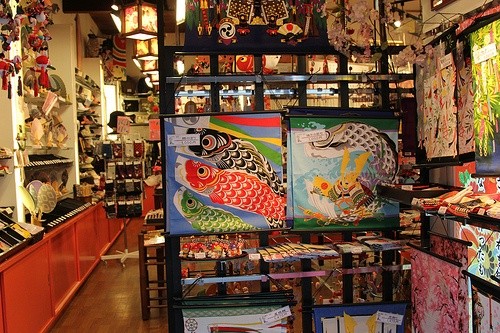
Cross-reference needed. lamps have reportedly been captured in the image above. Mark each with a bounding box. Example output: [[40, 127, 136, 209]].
[[117, 0, 158, 42], [132, 35, 159, 61], [140, 59, 160, 95]]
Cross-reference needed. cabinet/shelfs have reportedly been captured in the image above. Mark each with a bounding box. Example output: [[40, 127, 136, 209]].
[[158, 0, 499, 333], [1, 0, 136, 332]]
